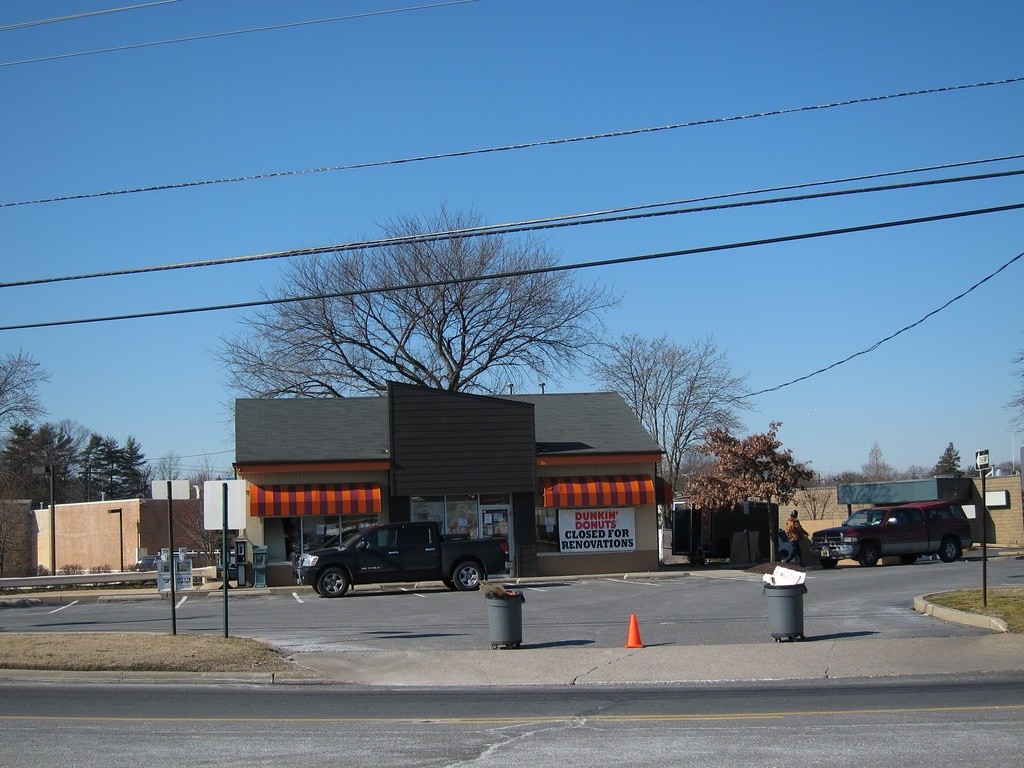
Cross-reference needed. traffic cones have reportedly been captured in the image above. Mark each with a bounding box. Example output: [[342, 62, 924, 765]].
[[625, 614, 644, 648]]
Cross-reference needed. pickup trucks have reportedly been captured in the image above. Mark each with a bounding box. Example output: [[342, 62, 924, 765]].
[[300, 521, 508, 598]]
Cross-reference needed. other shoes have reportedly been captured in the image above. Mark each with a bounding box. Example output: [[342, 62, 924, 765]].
[[782, 560, 788, 565]]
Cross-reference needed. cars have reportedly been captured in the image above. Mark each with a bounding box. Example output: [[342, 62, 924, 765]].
[[137, 557, 162, 570]]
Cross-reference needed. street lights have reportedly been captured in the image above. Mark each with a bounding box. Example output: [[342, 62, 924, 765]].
[[33, 464, 55, 576], [109, 508, 123, 572]]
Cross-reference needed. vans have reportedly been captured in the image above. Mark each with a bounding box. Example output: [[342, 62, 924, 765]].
[[811, 501, 971, 567]]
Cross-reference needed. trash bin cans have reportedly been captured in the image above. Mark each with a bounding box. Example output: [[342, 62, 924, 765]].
[[484, 589, 526, 650], [763, 581, 809, 644]]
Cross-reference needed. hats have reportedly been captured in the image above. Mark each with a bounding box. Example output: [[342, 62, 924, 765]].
[[790, 510, 798, 516]]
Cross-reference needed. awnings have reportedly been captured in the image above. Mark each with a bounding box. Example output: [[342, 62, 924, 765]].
[[538, 475, 655, 507], [657, 476, 673, 505], [250, 482, 382, 516]]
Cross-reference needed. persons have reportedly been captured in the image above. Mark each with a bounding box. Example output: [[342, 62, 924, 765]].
[[781, 509, 809, 566]]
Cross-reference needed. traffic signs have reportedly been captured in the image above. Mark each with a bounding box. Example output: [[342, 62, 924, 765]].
[[975, 449, 989, 471]]
